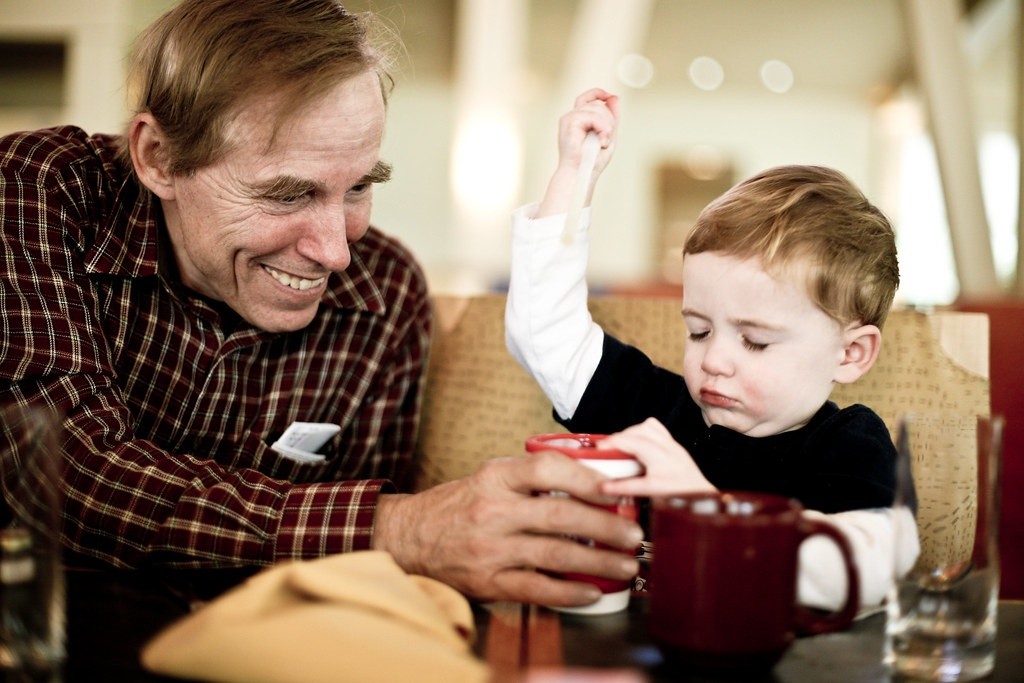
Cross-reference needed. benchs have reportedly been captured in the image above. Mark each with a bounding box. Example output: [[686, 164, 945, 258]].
[[416, 296, 1002, 586]]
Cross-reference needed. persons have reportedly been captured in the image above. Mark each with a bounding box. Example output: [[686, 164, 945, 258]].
[[0, 0, 643, 613], [500, 86, 923, 635]]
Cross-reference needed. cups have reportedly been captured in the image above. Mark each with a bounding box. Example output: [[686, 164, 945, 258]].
[[648, 496, 859, 669], [881, 415, 1004, 683], [527, 429, 642, 617], [1, 406, 66, 668]]
